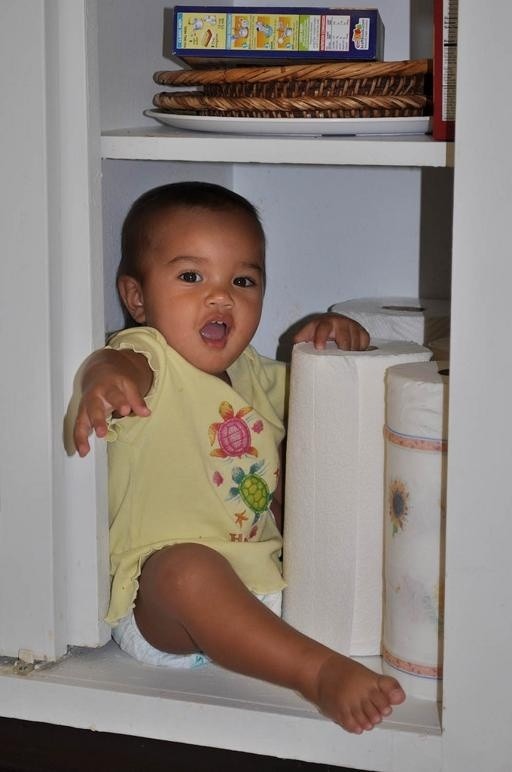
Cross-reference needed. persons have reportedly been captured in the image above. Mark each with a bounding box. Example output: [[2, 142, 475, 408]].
[[69, 181, 408, 736]]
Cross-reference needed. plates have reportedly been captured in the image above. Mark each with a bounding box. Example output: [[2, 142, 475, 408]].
[[145, 109, 433, 137]]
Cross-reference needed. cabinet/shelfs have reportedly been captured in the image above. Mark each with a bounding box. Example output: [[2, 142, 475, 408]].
[[72, 0, 453, 742]]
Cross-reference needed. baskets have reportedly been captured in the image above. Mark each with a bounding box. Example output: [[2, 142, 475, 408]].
[[152, 58, 433, 98], [151, 90, 430, 118]]
